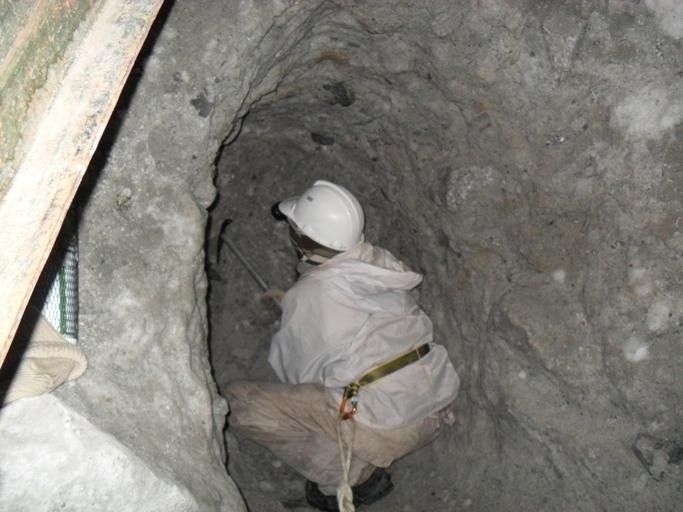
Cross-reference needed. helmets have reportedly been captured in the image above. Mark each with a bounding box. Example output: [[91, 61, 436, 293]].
[[278, 181, 365, 252]]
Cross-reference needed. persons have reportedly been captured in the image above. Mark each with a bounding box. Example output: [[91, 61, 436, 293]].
[[218, 179, 462, 512]]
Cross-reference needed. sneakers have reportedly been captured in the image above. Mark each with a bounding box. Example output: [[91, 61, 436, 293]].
[[306, 468, 393, 511]]
